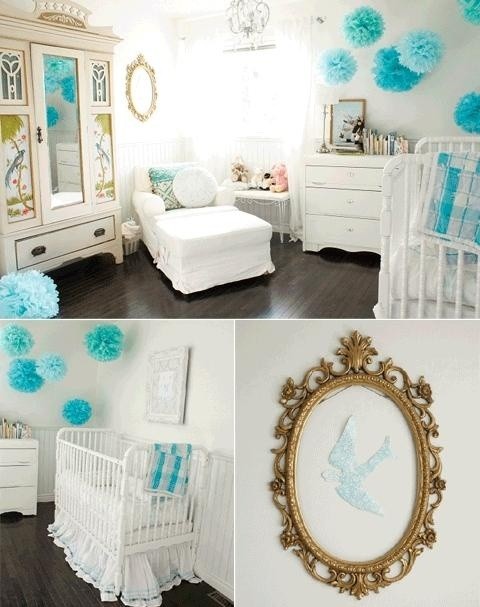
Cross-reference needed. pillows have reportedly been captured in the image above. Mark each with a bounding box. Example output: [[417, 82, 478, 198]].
[[174, 169, 217, 209], [147, 162, 200, 209]]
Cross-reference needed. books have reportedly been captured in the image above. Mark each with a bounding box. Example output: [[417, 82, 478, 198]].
[[0, 418, 32, 438], [363, 128, 409, 156]]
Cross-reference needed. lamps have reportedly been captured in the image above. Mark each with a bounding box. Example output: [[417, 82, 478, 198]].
[[315, 83, 339, 155], [222, 1, 275, 53]]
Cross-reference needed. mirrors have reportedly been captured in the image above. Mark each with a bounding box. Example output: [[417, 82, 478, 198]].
[[39, 48, 86, 211]]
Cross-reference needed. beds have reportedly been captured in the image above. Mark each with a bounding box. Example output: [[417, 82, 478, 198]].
[[48, 431, 210, 606], [374, 135, 480, 319]]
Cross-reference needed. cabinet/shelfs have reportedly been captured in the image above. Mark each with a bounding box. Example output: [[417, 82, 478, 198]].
[[234, 191, 291, 243], [1, 0, 123, 273]]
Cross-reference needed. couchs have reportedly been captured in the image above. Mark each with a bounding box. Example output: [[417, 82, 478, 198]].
[[132, 157, 274, 294]]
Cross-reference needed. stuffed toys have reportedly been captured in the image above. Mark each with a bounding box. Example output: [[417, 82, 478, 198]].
[[339, 115, 365, 145], [232, 155, 270, 190], [270, 162, 286, 192]]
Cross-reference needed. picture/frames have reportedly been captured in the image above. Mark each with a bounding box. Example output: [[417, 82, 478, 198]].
[[329, 98, 366, 152], [127, 53, 158, 121], [146, 346, 188, 426], [268, 330, 444, 598]]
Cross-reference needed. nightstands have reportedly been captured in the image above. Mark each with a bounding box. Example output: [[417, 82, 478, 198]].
[[0, 439, 39, 515], [304, 152, 392, 256]]
